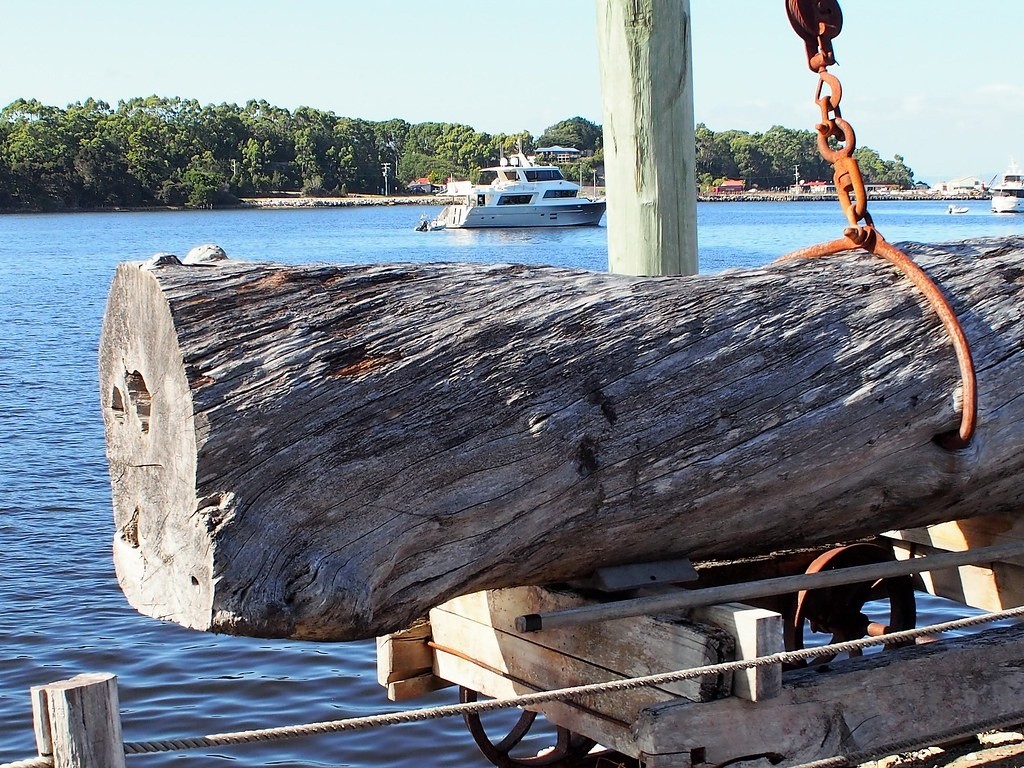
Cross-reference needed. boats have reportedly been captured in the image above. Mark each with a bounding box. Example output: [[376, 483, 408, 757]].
[[989, 169, 1024, 213], [413, 158, 608, 233], [946, 203, 969, 214]]
